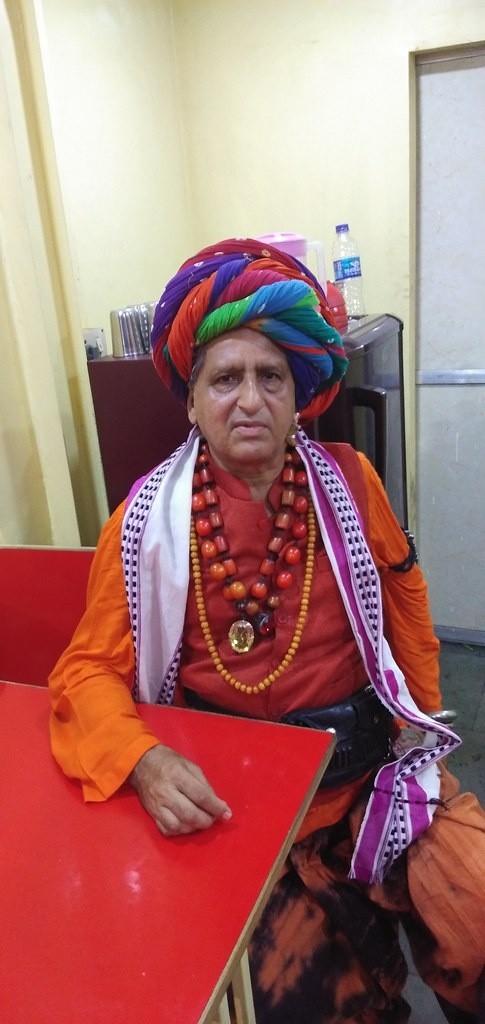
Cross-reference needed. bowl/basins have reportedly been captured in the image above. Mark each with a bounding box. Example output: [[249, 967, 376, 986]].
[[326, 279, 348, 334]]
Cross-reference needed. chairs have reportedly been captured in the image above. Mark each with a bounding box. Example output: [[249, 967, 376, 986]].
[[0, 545, 95, 688]]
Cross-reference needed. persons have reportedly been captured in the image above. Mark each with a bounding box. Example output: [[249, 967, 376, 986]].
[[46, 238, 485, 1024]]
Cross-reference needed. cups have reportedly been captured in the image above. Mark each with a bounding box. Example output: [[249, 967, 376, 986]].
[[110, 302, 157, 358]]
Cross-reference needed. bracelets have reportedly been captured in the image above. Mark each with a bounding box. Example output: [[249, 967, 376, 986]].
[[423, 710, 457, 727]]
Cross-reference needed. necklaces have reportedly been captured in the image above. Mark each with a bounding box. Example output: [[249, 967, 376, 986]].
[[188, 437, 316, 694]]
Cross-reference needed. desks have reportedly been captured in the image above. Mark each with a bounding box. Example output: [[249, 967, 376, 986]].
[[87, 356, 194, 519], [0, 683, 336, 1024]]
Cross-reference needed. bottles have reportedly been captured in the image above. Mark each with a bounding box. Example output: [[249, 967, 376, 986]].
[[332, 225, 364, 325]]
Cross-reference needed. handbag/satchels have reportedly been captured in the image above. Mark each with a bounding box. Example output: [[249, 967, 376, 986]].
[[285, 688, 394, 789]]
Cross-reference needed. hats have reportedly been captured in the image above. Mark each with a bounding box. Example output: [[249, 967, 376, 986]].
[[148, 237, 348, 423]]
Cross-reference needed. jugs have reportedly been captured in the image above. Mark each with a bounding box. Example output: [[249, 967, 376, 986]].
[[256, 232, 326, 283]]
